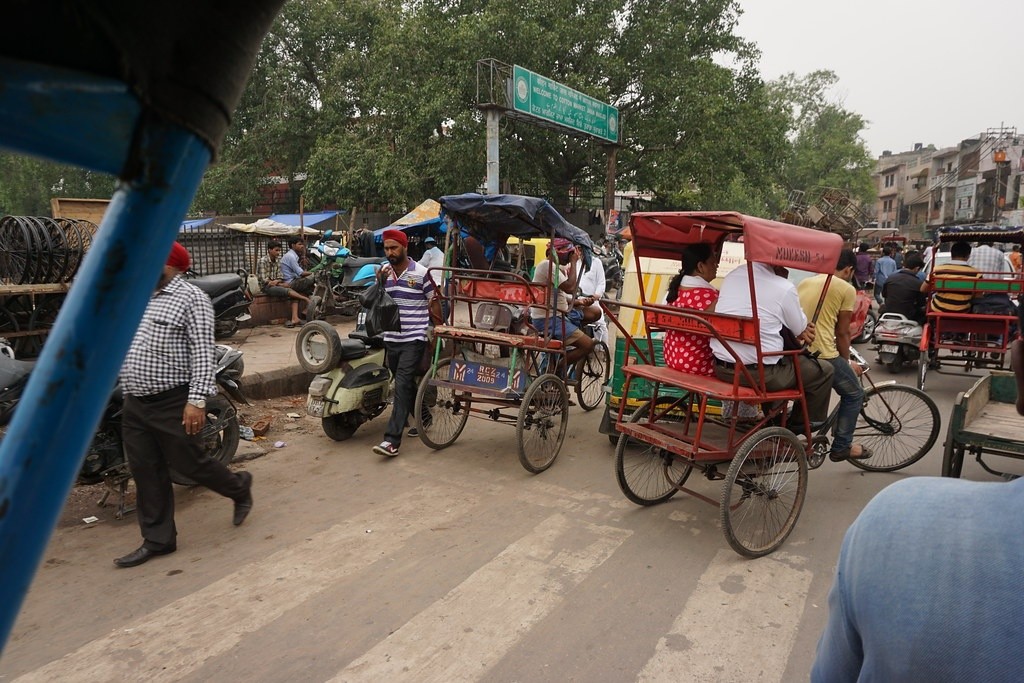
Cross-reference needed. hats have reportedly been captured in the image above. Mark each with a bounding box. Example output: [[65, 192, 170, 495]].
[[166, 241, 190, 271], [424, 237, 434, 242], [382, 229, 407, 248], [546, 238, 575, 253]]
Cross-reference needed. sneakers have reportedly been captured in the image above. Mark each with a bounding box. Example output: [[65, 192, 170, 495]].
[[373, 440, 398, 456], [407, 421, 430, 436]]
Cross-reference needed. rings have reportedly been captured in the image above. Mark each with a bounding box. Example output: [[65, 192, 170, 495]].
[[192, 422, 198, 425]]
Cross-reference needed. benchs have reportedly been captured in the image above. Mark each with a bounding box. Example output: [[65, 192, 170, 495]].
[[923, 270, 1024, 334], [623, 303, 801, 402], [434, 275, 562, 351]]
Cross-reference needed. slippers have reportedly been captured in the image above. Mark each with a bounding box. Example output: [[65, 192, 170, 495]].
[[294, 320, 305, 326], [284, 320, 294, 328]]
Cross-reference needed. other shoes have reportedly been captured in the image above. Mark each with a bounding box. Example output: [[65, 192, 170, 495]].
[[990, 344, 1000, 359], [555, 362, 562, 380], [568, 400, 576, 406], [787, 422, 824, 436]]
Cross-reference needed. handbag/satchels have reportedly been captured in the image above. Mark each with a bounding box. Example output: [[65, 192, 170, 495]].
[[360, 273, 402, 337]]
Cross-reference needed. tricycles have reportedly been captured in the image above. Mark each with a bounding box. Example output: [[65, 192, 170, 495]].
[[597, 210, 942, 559], [943, 370, 1024, 479], [918, 224, 1024, 390], [414, 193, 611, 474]]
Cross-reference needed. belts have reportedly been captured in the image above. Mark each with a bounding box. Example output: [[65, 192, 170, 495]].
[[715, 357, 754, 370]]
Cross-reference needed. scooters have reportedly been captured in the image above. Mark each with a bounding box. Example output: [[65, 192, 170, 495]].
[[306, 230, 413, 322], [296, 319, 437, 441]]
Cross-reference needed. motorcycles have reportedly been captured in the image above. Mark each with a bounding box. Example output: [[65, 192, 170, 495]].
[[181, 266, 256, 338], [0, 336, 250, 520]]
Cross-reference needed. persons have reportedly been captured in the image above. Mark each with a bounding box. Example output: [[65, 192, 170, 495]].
[[113, 242, 253, 567], [810, 293, 1024, 683], [874, 241, 1018, 369], [373, 230, 445, 457], [663, 242, 874, 462], [530, 233, 624, 414], [256, 237, 339, 326]]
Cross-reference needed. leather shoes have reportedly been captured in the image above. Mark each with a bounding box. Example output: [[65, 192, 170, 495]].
[[113, 545, 176, 566], [233, 471, 253, 525]]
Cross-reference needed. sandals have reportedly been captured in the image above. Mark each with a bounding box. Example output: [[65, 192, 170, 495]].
[[829, 444, 873, 462]]
